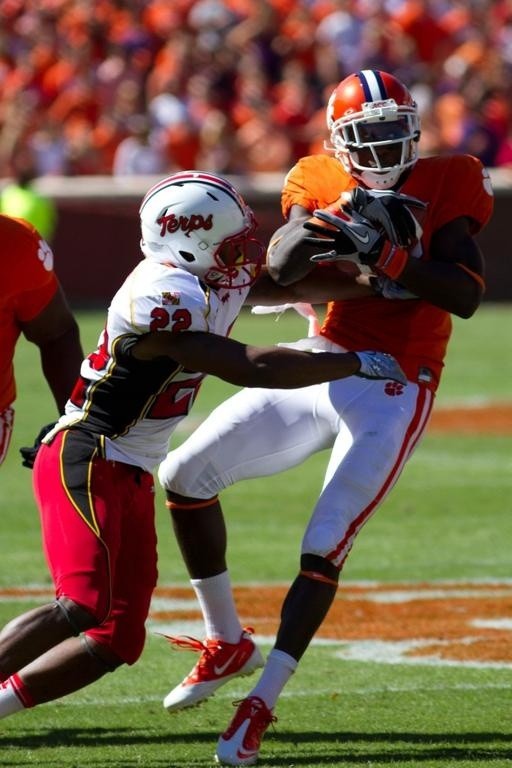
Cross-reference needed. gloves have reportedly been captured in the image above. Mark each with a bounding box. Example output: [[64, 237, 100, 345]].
[[371, 268, 424, 304], [342, 183, 431, 250], [350, 347, 411, 389], [17, 421, 60, 469], [300, 204, 403, 279]]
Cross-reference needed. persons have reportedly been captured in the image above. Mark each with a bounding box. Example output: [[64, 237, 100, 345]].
[[0, 169, 409, 721], [0, 215, 85, 470], [158, 70, 496, 767]]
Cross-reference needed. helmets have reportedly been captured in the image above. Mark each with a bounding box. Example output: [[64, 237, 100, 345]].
[[137, 170, 258, 279], [324, 67, 422, 191]]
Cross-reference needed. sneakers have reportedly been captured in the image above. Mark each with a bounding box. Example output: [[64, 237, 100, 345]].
[[216, 695, 279, 767], [165, 628, 265, 715]]
